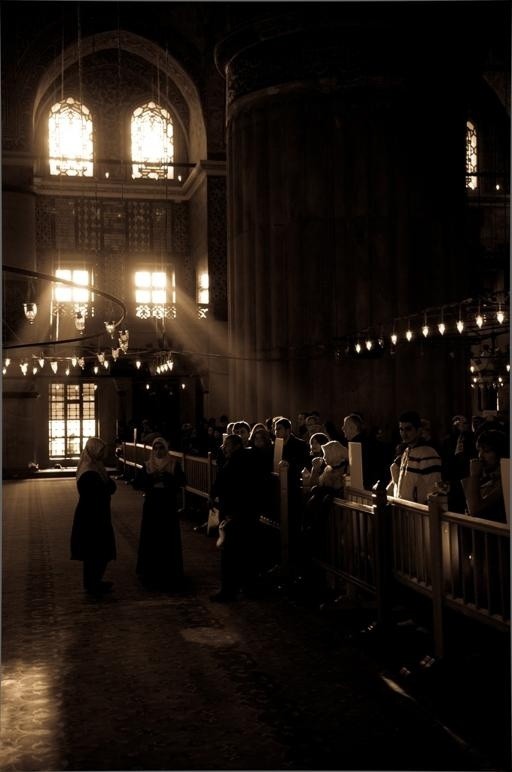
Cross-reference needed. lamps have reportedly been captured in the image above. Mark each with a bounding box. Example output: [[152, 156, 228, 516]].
[[405, 319, 414, 343], [4, 348, 12, 369], [494, 295, 507, 326], [75, 287, 87, 335], [354, 332, 364, 356], [474, 301, 485, 330], [421, 312, 431, 339], [378, 325, 387, 349], [391, 323, 399, 347], [37, 350, 49, 369], [49, 361, 59, 375], [437, 310, 448, 336], [96, 338, 108, 366], [111, 334, 120, 362], [455, 305, 466, 336], [364, 328, 375, 352], [119, 318, 131, 354], [24, 276, 39, 324], [151, 354, 175, 377], [65, 360, 73, 378], [104, 313, 118, 334]]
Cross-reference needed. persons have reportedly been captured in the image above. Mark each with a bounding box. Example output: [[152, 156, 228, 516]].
[[302, 433, 329, 480], [342, 414, 380, 489], [132, 437, 186, 579], [306, 441, 348, 502], [470, 412, 510, 430], [272, 416, 282, 431], [227, 423, 234, 434], [139, 416, 228, 453], [210, 435, 264, 567], [298, 413, 327, 435], [390, 421, 433, 487], [251, 423, 267, 434], [397, 412, 444, 504], [275, 418, 309, 481], [462, 430, 510, 523], [69, 436, 117, 599], [449, 416, 470, 457], [249, 429, 274, 474], [231, 422, 250, 448]]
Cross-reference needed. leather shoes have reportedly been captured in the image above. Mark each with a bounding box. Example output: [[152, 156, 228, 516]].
[[98, 581, 113, 588], [88, 587, 106, 595], [239, 587, 255, 593], [209, 592, 235, 604]]
